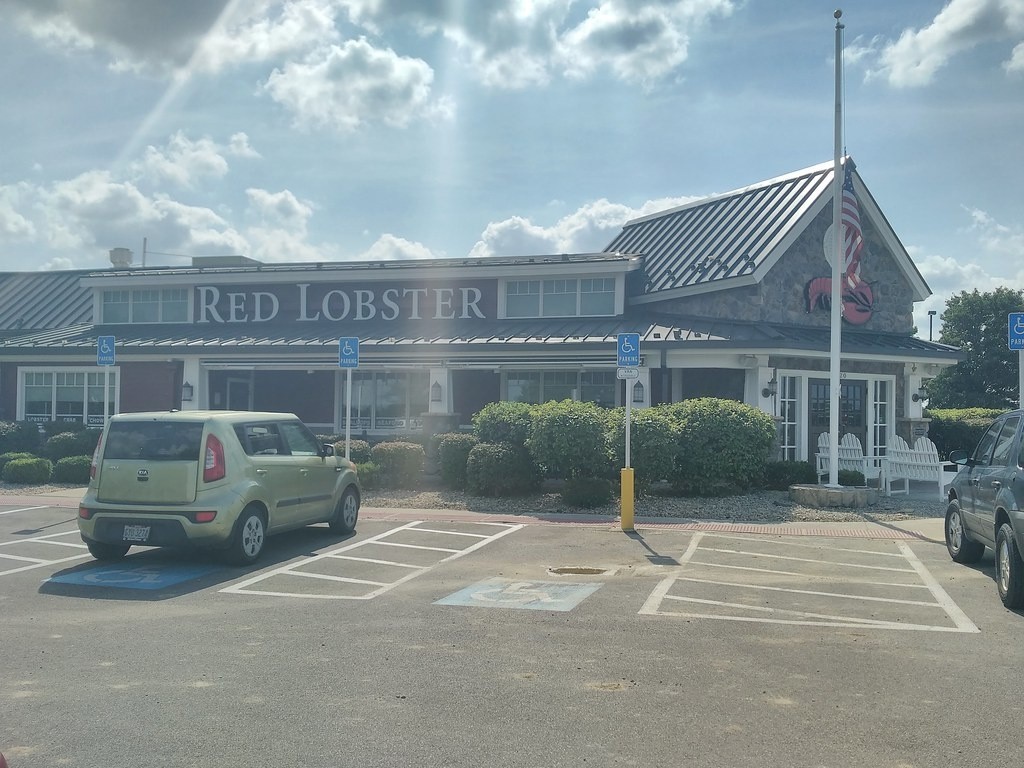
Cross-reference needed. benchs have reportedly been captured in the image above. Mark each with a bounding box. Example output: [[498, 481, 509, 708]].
[[886, 435, 957, 502], [813, 432, 886, 492]]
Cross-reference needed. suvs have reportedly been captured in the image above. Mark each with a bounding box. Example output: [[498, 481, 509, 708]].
[[942, 409, 1024, 612]]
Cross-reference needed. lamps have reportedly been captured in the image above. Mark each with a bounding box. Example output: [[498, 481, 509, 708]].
[[431, 381, 441, 402], [181, 381, 193, 401], [633, 381, 644, 402], [762, 378, 778, 398], [912, 385, 927, 402]]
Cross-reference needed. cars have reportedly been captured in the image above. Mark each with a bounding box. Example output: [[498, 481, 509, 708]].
[[75, 406, 365, 570]]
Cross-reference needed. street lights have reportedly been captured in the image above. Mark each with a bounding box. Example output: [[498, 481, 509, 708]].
[[927, 310, 937, 404]]
